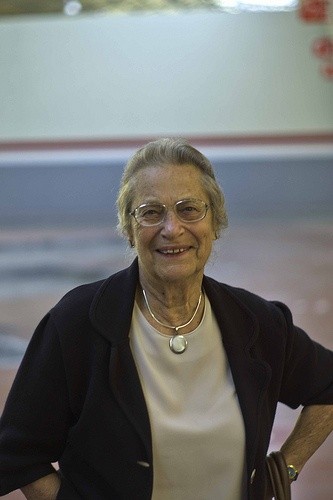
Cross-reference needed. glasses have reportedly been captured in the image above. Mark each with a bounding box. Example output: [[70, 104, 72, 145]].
[[127, 198, 212, 227]]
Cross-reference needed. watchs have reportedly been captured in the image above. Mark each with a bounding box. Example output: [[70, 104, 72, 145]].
[[285, 464, 299, 481]]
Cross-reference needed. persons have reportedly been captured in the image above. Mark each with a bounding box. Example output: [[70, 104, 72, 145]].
[[0, 138, 333, 500]]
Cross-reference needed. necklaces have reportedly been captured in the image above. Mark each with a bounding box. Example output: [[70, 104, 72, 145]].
[[142, 290, 201, 354]]
